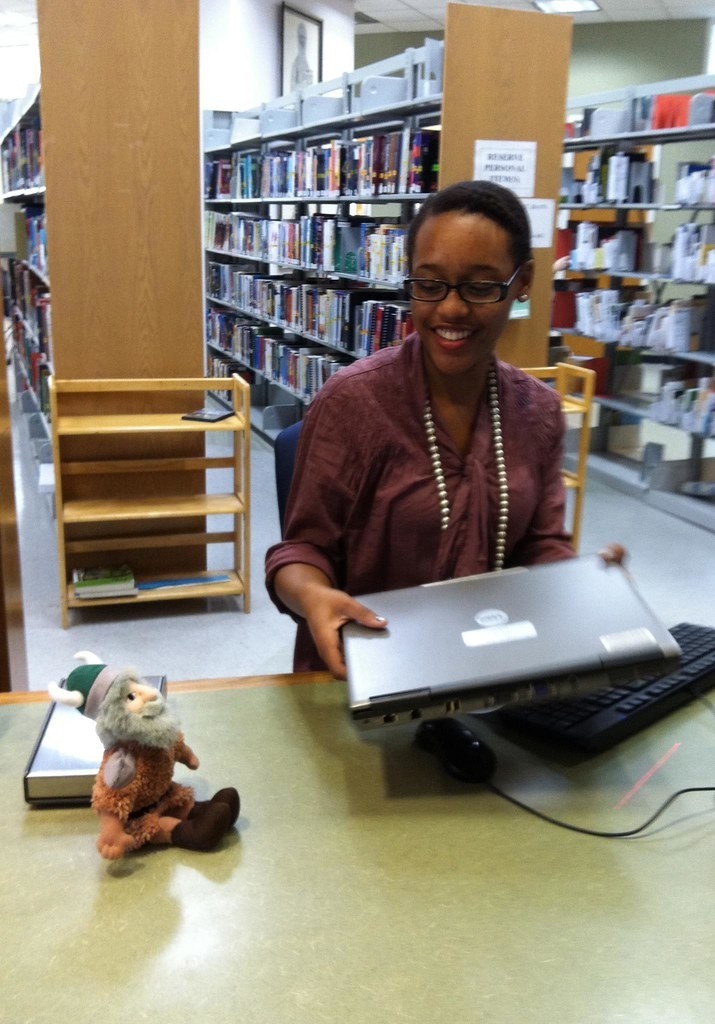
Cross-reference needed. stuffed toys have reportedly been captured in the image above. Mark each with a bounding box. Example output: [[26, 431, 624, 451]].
[[46, 651, 240, 859]]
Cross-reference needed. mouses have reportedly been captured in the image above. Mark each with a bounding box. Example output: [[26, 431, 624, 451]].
[[413, 716, 495, 783]]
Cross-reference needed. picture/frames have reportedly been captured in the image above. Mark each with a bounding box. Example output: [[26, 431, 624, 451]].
[[279, 1, 324, 97]]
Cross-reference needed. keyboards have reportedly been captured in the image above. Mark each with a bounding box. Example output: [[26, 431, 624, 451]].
[[487, 621, 715, 762]]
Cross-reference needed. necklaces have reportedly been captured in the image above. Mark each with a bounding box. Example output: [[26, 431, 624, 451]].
[[421, 363, 509, 573]]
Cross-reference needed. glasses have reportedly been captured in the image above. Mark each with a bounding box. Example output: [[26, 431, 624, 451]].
[[400, 265, 522, 304]]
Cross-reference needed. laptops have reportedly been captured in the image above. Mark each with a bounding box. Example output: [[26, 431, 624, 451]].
[[343, 556, 681, 719]]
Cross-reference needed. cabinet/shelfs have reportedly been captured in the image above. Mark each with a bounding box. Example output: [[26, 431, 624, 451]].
[[202, 1, 575, 448], [47, 370, 252, 627], [0, 0, 209, 583], [519, 360, 598, 553], [555, 73, 715, 526]]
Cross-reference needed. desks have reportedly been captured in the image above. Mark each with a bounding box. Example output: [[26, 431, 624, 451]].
[[0, 670, 715, 1024]]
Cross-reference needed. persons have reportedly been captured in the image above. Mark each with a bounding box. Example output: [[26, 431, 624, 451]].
[[551, 255, 570, 275], [263, 172, 631, 677]]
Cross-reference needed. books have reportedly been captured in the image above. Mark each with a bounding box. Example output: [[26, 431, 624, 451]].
[[22, 675, 167, 807], [555, 91, 715, 497], [74, 562, 138, 600], [1, 124, 57, 495], [184, 407, 233, 422], [203, 121, 440, 402]]
